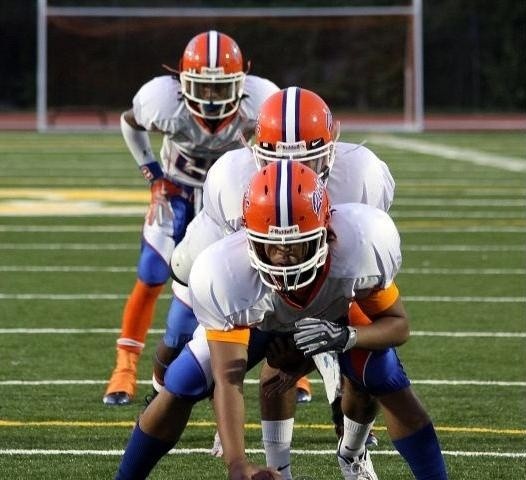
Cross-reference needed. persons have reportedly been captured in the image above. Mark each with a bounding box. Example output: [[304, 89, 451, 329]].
[[143, 86, 395, 480], [115, 160, 448, 480], [103, 30, 282, 404]]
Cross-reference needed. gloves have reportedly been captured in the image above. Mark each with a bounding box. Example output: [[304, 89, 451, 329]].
[[139, 178, 180, 226], [293, 316, 357, 357], [261, 369, 298, 401]]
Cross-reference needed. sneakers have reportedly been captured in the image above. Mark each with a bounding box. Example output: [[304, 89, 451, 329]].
[[295, 377, 312, 403], [337, 436, 378, 480], [103, 365, 137, 404], [211, 432, 224, 457], [337, 429, 377, 449]]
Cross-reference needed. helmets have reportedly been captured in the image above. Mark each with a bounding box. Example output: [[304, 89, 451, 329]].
[[252, 86, 340, 187], [243, 159, 334, 293], [178, 30, 245, 135]]
